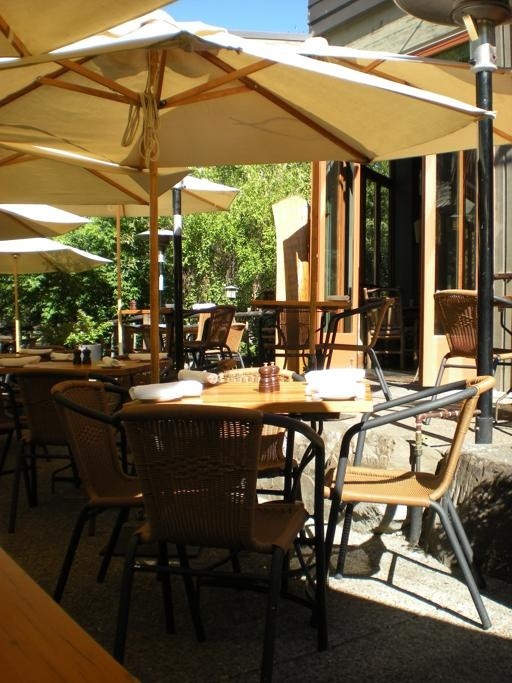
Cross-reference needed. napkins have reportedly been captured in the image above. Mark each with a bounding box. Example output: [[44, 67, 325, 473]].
[[178, 369, 218, 384], [303, 369, 365, 384], [129, 379, 202, 400]]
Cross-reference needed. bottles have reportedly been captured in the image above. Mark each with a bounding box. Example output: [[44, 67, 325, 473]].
[[270, 361, 281, 391], [83, 346, 92, 364], [73, 345, 82, 364], [258, 361, 273, 392]]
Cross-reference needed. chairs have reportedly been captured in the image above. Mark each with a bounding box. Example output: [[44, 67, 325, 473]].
[[52, 379, 146, 605], [1, 336, 170, 533], [323, 376, 492, 631], [217, 366, 306, 546], [113, 293, 395, 403], [113, 406, 326, 681], [424, 288, 510, 427], [365, 286, 419, 368]]
